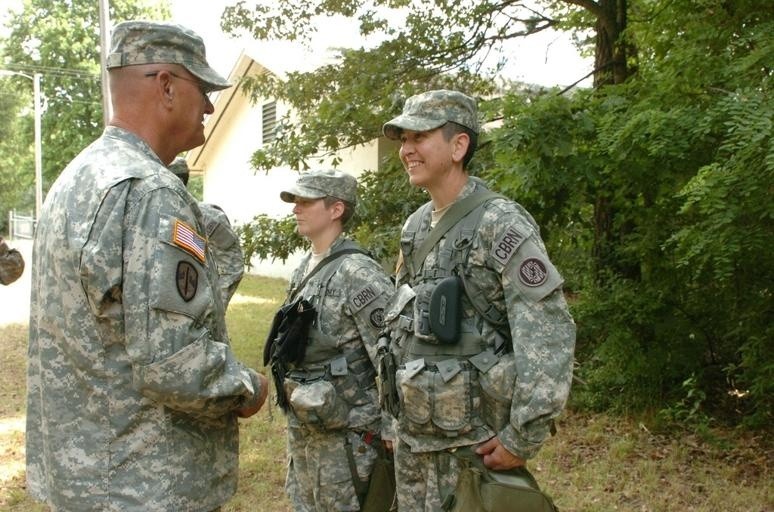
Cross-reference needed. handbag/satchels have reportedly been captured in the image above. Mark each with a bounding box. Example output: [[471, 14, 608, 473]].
[[290, 382, 350, 434], [479, 353, 514, 430], [361, 456, 396, 512], [447, 468, 552, 511], [397, 359, 471, 438]]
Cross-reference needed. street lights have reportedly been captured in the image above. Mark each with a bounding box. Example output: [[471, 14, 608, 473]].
[[0, 70, 42, 223]]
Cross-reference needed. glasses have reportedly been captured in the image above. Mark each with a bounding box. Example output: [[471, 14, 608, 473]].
[[144, 70, 211, 99]]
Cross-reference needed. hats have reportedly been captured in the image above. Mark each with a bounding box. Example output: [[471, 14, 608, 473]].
[[167, 156, 189, 174], [383, 89, 478, 140], [106, 22, 233, 90], [280, 171, 358, 203]]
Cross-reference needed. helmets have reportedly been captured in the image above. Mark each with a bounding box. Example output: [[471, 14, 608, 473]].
[[1, 248, 22, 284]]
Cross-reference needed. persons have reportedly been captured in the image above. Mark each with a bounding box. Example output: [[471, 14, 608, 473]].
[[26, 21, 268, 511], [263, 169, 395, 511], [373, 90, 577, 512]]
